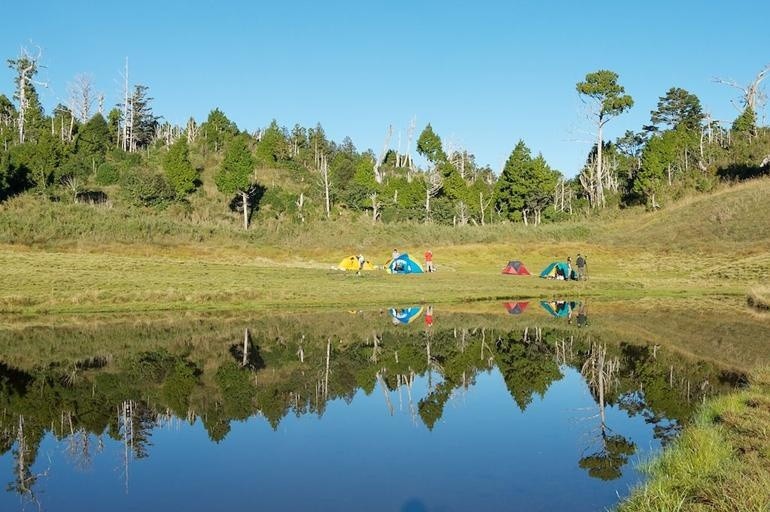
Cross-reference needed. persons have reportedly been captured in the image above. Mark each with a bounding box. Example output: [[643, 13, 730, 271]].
[[575, 253, 587, 281], [392, 249, 399, 259], [356, 255, 365, 276], [567, 256, 573, 281], [425, 248, 434, 273], [392, 317, 400, 327], [425, 305, 433, 328], [567, 301, 576, 324], [575, 301, 587, 329]]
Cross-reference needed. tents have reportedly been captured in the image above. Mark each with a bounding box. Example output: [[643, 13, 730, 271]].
[[337, 254, 377, 271], [502, 302, 530, 314], [388, 307, 424, 325], [502, 260, 531, 275], [540, 300, 577, 318], [383, 251, 425, 274], [540, 260, 578, 280]]
